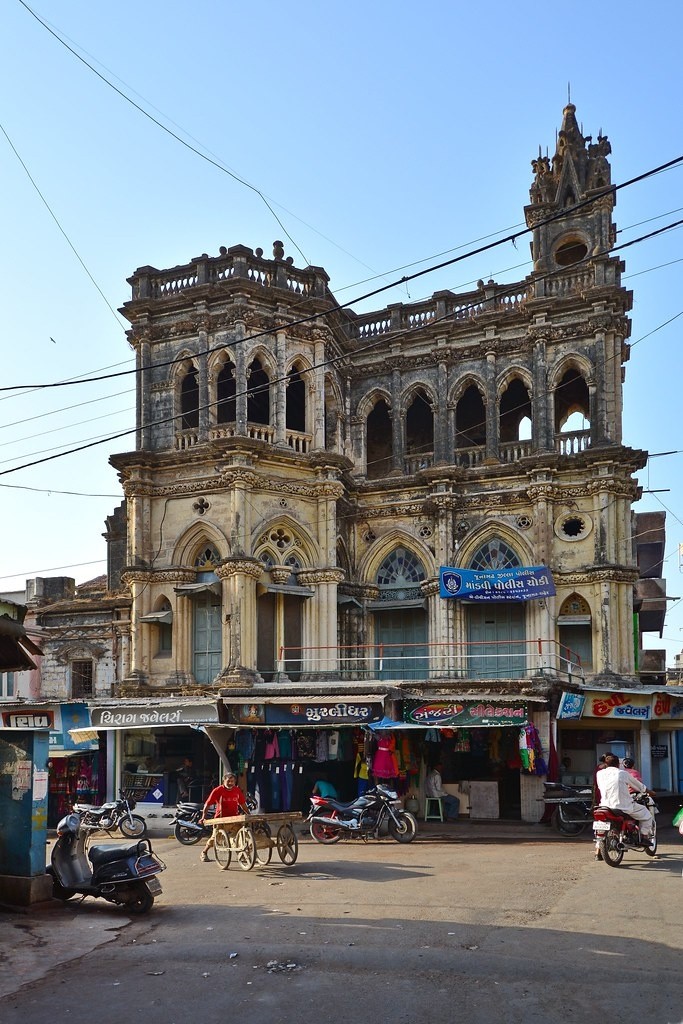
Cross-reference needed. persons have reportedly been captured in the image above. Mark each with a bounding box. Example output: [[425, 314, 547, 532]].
[[622, 758, 643, 804], [312, 776, 338, 802], [424, 762, 461, 825], [592, 752, 614, 809], [175, 757, 199, 806], [594, 755, 655, 862], [199, 772, 251, 862]]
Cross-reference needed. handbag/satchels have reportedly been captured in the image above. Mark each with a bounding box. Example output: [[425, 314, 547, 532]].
[[673, 808, 683, 835]]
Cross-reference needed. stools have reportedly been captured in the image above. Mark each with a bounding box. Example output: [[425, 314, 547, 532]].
[[424, 798, 443, 822]]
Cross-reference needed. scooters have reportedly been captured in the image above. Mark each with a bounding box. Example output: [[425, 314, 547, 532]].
[[47, 812, 167, 914]]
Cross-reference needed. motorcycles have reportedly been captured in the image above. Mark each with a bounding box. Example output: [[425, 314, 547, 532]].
[[543, 782, 594, 836], [303, 784, 419, 845], [592, 791, 658, 868], [168, 792, 259, 845], [72, 787, 147, 838]]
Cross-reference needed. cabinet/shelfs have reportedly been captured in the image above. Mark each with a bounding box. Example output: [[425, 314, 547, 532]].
[[121, 773, 164, 803]]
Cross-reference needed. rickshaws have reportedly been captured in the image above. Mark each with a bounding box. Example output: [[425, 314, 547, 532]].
[[199, 809, 305, 870]]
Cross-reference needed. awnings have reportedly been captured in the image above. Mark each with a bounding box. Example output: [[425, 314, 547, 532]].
[[255, 582, 314, 605], [172, 581, 222, 597], [139, 610, 174, 625]]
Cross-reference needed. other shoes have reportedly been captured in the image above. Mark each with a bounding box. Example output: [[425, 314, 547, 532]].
[[182, 793, 188, 798], [200, 851, 210, 861], [641, 839, 654, 846], [595, 854, 603, 861]]
